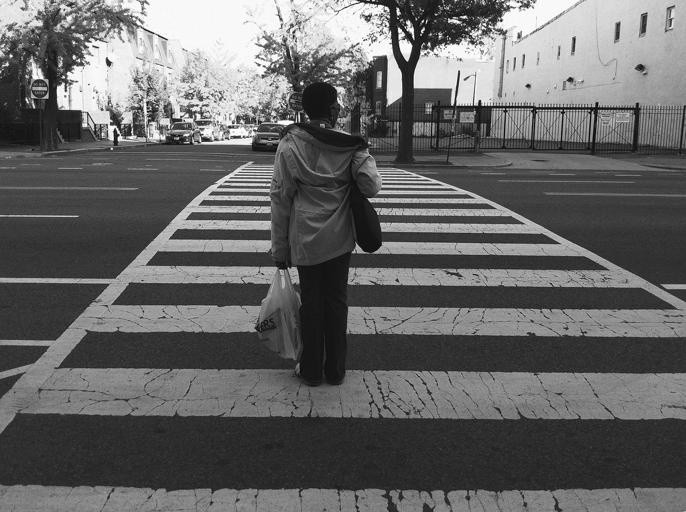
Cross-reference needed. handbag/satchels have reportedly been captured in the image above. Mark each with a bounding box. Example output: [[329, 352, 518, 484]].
[[350, 161, 382, 254]]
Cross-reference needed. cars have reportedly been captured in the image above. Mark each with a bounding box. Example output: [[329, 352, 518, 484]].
[[276, 119, 294, 128], [164, 121, 202, 144], [194, 117, 258, 142], [251, 123, 285, 152]]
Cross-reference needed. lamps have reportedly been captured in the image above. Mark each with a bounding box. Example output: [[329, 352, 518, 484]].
[[525, 84, 530, 88], [567, 77, 573, 82], [635, 64, 644, 71]]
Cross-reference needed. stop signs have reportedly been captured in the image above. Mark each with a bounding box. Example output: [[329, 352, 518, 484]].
[[29, 77, 49, 99]]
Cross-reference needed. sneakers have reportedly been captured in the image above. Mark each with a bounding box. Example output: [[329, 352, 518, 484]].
[[295, 363, 345, 386]]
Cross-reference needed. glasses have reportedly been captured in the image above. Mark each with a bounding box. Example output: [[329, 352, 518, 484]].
[[331, 104, 340, 112]]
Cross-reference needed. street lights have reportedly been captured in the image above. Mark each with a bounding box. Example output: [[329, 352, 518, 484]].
[[464, 69, 476, 115]]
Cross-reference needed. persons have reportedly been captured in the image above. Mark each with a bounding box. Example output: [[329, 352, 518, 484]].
[[269, 82, 382, 386]]
